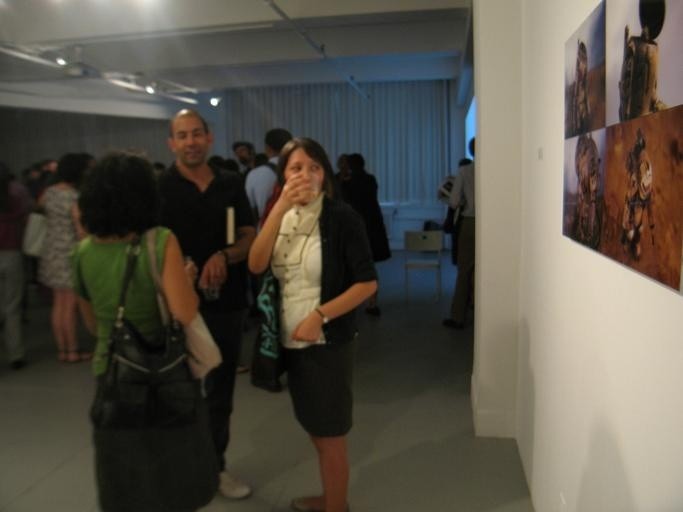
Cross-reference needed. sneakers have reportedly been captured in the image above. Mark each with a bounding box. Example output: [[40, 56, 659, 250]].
[[218, 471, 250, 498]]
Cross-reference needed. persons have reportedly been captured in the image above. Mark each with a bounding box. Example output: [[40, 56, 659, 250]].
[[207, 141, 393, 344], [243, 128, 300, 395], [67, 152, 221, 512], [247, 138, 378, 512], [442, 138, 475, 329], [151, 108, 256, 501], [2, 153, 167, 370]]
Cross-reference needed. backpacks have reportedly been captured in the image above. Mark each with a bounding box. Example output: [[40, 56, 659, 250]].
[[261, 162, 286, 231]]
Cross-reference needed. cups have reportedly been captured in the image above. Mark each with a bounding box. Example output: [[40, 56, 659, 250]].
[[300, 174, 319, 201]]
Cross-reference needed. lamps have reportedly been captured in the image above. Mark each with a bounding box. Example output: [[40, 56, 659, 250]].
[[145, 81, 156, 95], [54, 49, 69, 65], [210, 94, 224, 108]]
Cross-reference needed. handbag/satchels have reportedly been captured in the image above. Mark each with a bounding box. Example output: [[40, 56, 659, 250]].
[[250, 273, 288, 392], [90, 317, 201, 430], [23, 213, 48, 257]]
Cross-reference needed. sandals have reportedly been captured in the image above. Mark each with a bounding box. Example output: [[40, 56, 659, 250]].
[[57, 349, 90, 363], [292, 496, 348, 511]]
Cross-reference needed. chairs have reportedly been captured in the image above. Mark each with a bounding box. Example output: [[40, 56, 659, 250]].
[[402, 231, 443, 305]]
